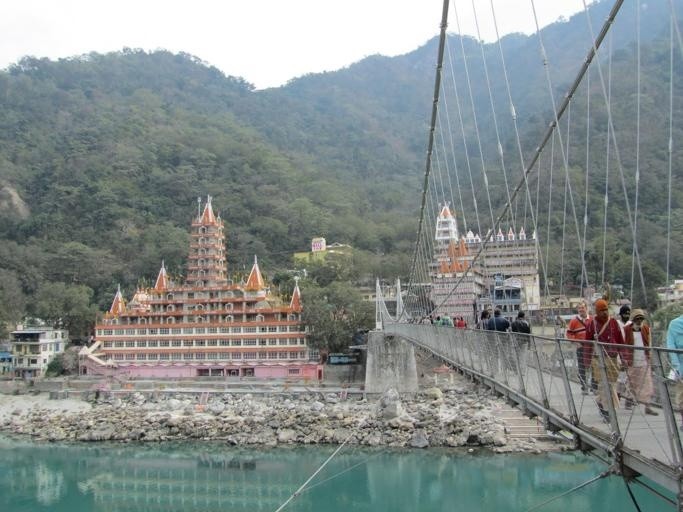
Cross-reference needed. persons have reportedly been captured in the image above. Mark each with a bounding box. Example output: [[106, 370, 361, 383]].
[[406, 293, 683, 434]]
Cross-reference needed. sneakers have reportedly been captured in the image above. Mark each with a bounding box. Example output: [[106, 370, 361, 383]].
[[625, 400, 632, 409], [645, 408, 659, 415]]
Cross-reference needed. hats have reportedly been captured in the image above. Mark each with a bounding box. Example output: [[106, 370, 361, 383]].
[[596, 300, 608, 315], [619, 307, 630, 315], [630, 309, 645, 321]]
